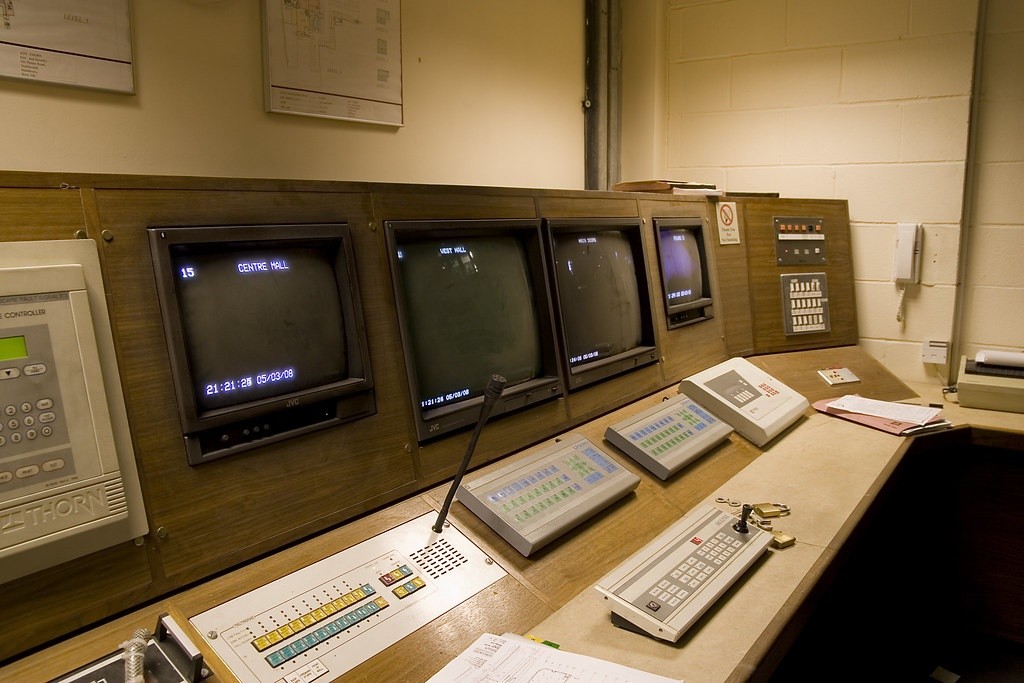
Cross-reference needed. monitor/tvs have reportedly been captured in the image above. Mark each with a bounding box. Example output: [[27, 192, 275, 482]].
[[652, 217, 712, 316], [542, 217, 660, 391], [383, 218, 565, 441], [148, 224, 374, 435]]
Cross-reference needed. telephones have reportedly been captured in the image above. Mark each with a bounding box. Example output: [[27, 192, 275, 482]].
[[892, 222, 924, 284]]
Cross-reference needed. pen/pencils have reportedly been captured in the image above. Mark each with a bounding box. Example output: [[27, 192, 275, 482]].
[[895, 402, 922, 405]]
